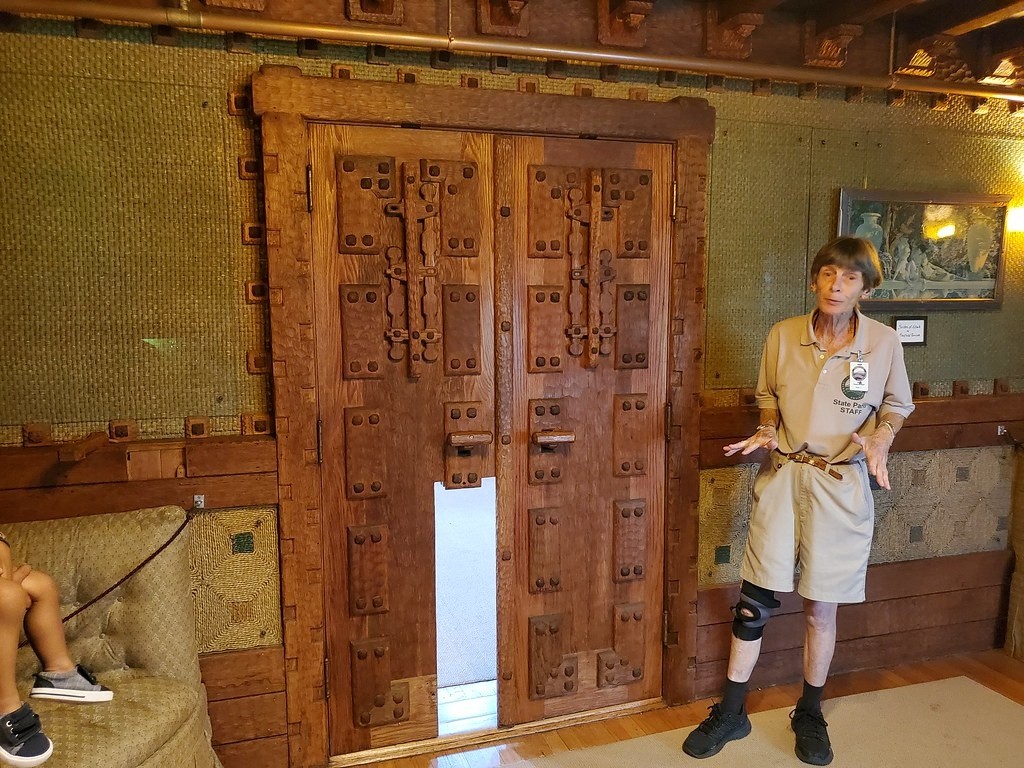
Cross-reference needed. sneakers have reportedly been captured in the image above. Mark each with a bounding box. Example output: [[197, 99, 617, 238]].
[[790, 697, 835, 766], [1, 701, 54, 767], [681, 700, 752, 759], [28, 664, 114, 703]]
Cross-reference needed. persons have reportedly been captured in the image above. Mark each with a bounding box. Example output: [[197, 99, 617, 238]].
[[678, 235, 918, 768], [0, 531, 115, 768]]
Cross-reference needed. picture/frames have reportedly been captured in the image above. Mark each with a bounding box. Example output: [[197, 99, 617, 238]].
[[838, 186, 1014, 311], [894, 315, 928, 346]]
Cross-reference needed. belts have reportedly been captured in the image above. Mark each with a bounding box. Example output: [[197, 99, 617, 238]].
[[777, 449, 866, 480]]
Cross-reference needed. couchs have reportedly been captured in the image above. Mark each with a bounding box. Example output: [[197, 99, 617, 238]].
[[0, 505, 223, 768]]
